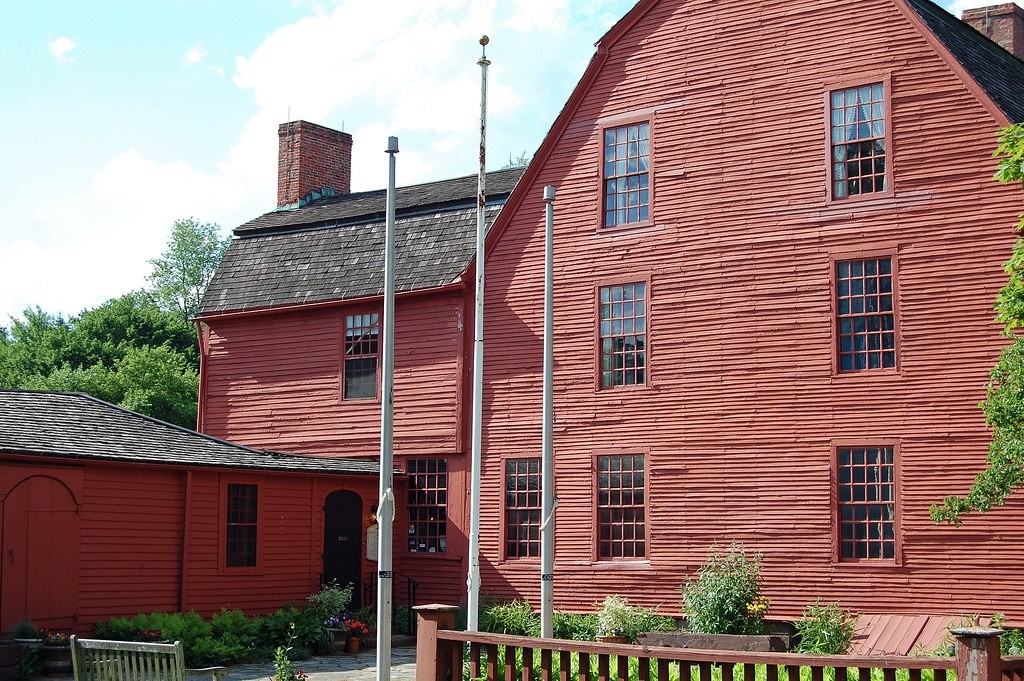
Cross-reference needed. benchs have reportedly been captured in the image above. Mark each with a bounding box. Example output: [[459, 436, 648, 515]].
[[70, 634, 229, 681]]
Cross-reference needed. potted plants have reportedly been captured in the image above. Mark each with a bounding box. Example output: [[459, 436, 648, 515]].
[[11, 617, 45, 652]]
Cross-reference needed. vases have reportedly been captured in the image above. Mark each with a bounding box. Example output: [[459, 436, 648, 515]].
[[38, 646, 72, 675], [347, 637, 361, 653], [326, 627, 351, 656], [595, 633, 628, 644]]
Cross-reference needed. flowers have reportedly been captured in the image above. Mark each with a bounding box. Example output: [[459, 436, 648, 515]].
[[323, 610, 353, 629], [43, 629, 70, 646], [343, 619, 369, 638], [595, 620, 625, 636]]
